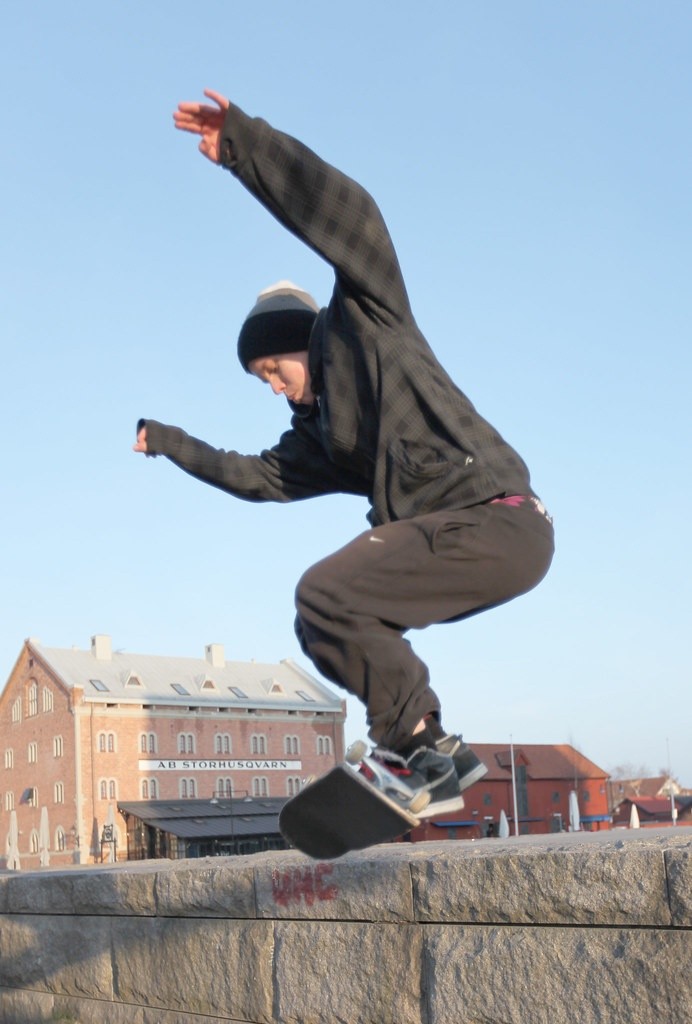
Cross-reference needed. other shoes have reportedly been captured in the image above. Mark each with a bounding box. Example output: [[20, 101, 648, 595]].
[[435, 734, 488, 793], [358, 746, 465, 818]]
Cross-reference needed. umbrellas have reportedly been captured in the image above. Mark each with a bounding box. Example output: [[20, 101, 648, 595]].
[[38, 806, 51, 868], [498, 789, 642, 840], [6, 810, 22, 873]]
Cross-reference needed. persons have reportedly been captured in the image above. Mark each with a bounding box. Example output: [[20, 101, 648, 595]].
[[131, 91, 555, 818], [486, 824, 496, 837]]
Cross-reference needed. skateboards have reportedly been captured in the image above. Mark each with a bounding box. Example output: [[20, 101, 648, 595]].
[[276, 741, 431, 858]]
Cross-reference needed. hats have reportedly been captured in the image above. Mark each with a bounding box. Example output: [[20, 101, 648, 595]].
[[238, 281, 319, 373]]
